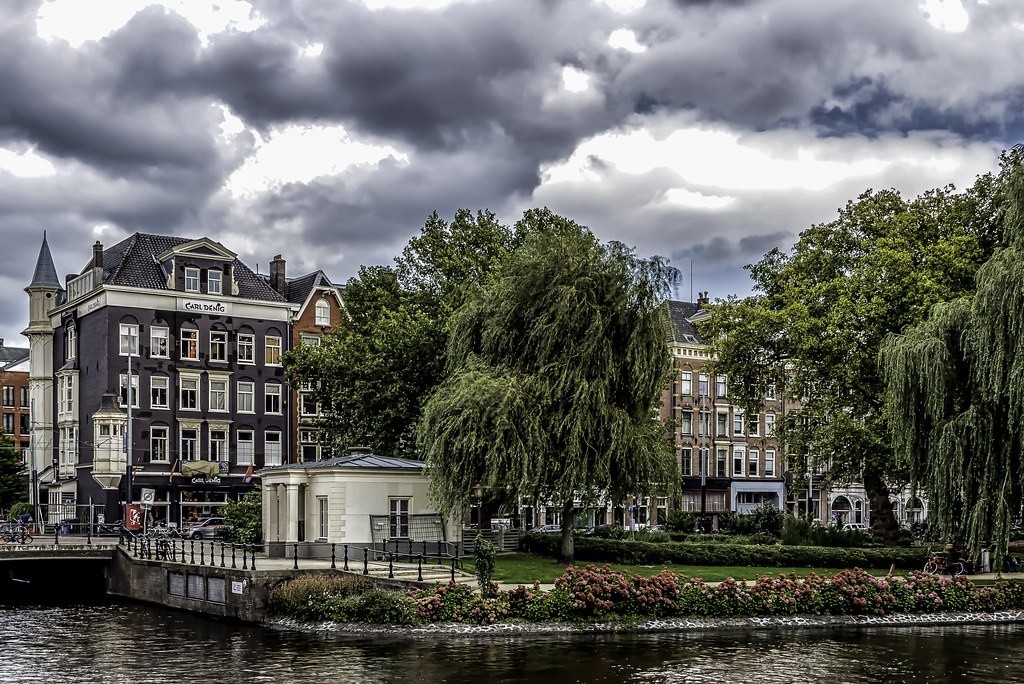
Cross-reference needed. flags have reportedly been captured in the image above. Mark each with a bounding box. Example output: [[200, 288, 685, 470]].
[[242, 463, 252, 484]]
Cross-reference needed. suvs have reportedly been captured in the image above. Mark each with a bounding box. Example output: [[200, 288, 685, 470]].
[[842, 523, 866, 532], [180, 517, 234, 541], [529, 525, 562, 535]]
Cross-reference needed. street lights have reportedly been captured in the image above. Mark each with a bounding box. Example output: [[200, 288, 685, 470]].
[[471, 484, 488, 535]]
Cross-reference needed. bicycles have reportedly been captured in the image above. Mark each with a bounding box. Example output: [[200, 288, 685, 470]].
[[923, 551, 964, 575], [0, 518, 34, 544], [137, 532, 173, 561]]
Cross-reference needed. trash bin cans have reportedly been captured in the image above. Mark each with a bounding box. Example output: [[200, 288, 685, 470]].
[[981, 548, 991, 572], [61, 519, 80, 533]]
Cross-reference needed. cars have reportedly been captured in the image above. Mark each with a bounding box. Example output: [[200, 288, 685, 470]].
[[640, 525, 674, 533], [584, 527, 596, 538]]
